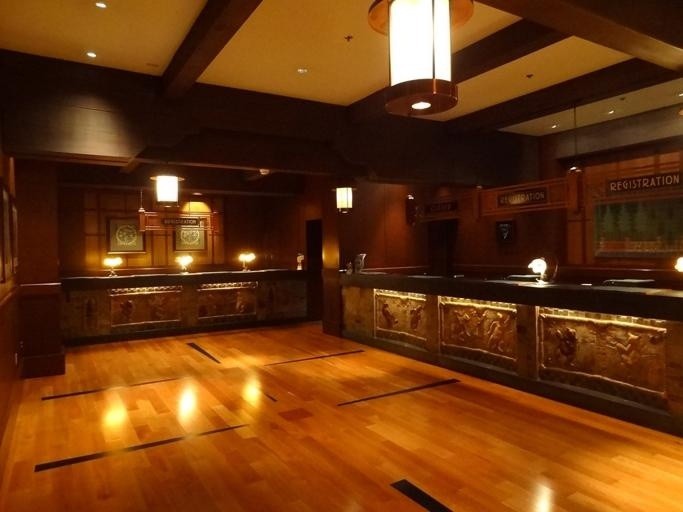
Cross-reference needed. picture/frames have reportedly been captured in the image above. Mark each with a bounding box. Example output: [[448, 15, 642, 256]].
[[174, 218, 208, 253], [106, 216, 147, 255]]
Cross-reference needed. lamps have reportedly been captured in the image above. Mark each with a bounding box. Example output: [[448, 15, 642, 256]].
[[239, 253, 256, 272], [526, 253, 560, 285], [150, 168, 185, 207], [104, 257, 122, 277], [331, 179, 358, 215], [368, 0, 474, 116], [177, 256, 193, 274]]
